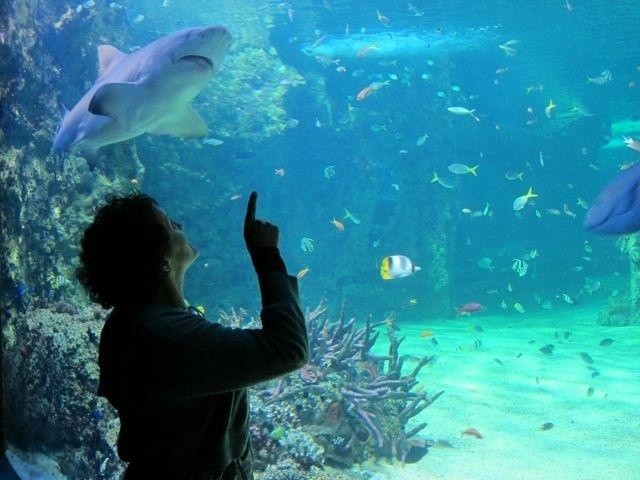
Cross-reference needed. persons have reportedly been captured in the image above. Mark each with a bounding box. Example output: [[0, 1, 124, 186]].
[[75, 190, 310, 480]]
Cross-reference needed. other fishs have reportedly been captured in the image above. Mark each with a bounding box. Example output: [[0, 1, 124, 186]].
[[190, 0, 639, 450]]
